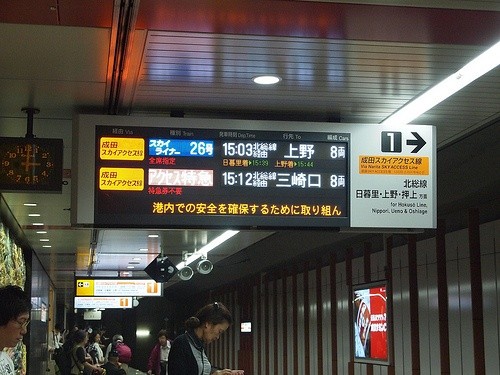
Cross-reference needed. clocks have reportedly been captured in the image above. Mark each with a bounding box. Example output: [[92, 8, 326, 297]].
[[0, 138, 63, 194]]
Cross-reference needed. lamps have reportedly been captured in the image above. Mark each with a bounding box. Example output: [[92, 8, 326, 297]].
[[251, 74, 282, 84]]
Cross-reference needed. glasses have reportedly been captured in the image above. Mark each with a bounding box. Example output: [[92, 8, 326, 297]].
[[14, 318, 30, 328]]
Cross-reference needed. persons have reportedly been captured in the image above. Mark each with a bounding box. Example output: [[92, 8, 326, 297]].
[[106, 334, 123, 360], [115, 339, 132, 364], [147, 333, 173, 375], [102, 352, 126, 375], [0, 285, 32, 375], [168, 302, 243, 375], [48, 324, 104, 375]]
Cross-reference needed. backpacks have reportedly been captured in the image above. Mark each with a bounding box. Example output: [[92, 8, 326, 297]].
[[54, 345, 81, 375]]
